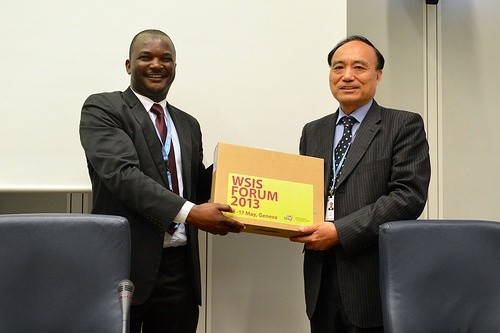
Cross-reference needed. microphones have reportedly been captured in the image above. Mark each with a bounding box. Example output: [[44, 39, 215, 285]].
[[118, 278, 135, 333]]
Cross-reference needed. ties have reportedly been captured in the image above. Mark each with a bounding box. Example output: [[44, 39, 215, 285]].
[[150, 104, 180, 235], [327, 116, 360, 195]]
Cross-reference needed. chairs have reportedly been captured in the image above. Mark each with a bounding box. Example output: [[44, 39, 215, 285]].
[[0, 213, 131, 333], [378, 218, 500, 333]]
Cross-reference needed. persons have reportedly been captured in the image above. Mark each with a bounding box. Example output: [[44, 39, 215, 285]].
[[288, 34, 431, 333], [78, 30, 247, 333]]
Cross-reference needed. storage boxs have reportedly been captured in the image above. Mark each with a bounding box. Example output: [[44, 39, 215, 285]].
[[212, 143, 326, 240]]
